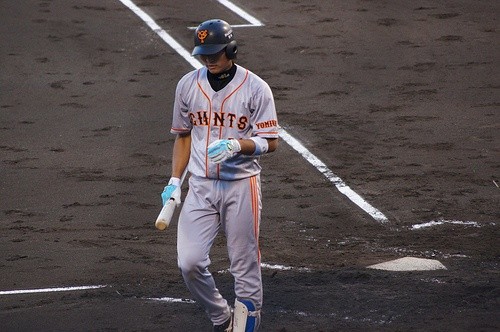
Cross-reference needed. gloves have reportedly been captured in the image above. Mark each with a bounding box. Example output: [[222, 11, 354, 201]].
[[160, 177, 182, 207], [206, 137, 241, 163]]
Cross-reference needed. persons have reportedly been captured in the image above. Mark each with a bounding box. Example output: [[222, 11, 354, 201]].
[[161, 19, 280, 332]]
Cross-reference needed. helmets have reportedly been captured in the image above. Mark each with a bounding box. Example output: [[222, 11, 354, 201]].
[[191, 18, 240, 59]]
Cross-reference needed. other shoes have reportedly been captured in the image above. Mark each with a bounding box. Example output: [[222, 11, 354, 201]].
[[213, 316, 234, 332]]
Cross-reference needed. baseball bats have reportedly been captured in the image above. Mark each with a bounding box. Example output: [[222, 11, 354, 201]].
[[154, 165, 189, 231]]
